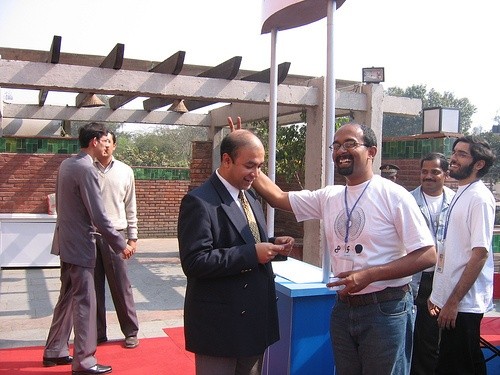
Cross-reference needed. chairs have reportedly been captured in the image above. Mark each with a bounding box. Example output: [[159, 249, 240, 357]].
[[480, 272, 500, 362]]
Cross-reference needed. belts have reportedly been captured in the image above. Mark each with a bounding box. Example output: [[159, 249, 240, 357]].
[[338, 285, 408, 306]]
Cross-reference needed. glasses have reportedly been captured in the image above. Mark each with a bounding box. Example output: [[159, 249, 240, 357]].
[[329, 142, 364, 151]]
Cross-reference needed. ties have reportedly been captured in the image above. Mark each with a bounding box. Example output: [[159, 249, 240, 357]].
[[238, 189, 260, 243]]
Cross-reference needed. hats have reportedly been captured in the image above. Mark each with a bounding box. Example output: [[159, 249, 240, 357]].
[[380, 164, 400, 177]]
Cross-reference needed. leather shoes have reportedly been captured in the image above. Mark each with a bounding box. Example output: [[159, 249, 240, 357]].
[[72, 364, 112, 375], [125, 337, 139, 347], [43, 356, 73, 366]]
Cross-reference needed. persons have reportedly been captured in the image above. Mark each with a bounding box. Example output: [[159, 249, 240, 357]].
[[177, 129, 295, 375], [94, 129, 139, 347], [227, 116, 496, 374], [42, 121, 134, 375]]
